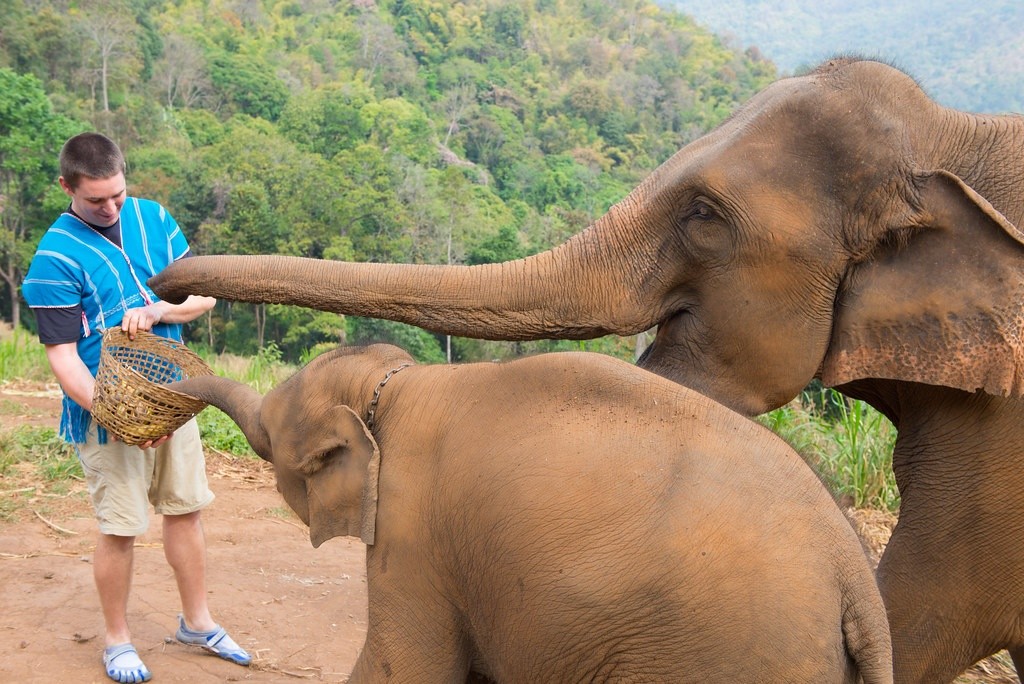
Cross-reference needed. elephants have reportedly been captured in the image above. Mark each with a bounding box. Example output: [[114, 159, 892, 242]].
[[138, 53, 1024, 684]]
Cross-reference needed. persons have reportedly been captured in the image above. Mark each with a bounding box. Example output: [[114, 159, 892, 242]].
[[20, 129, 252, 684]]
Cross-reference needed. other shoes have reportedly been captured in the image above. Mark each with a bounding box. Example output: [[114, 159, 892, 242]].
[[176, 618, 253, 665], [101, 643, 151, 684]]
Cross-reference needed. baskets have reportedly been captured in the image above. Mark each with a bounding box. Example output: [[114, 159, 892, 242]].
[[90, 327, 215, 447]]
